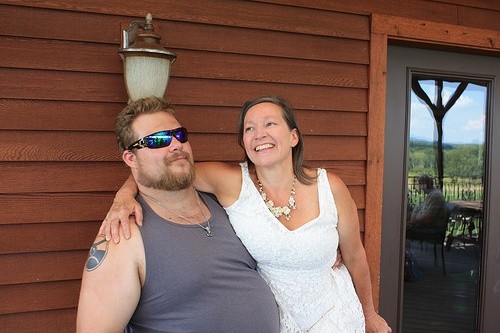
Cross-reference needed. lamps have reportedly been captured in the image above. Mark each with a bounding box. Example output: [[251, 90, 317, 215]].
[[117, 13, 176, 104]]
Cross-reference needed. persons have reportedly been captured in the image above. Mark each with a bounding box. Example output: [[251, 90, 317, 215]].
[[97, 96, 394, 333], [75, 95, 343, 333], [406, 174, 449, 242]]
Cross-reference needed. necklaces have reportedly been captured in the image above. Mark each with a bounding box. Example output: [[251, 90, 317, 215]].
[[254, 165, 298, 221], [140, 187, 214, 237]]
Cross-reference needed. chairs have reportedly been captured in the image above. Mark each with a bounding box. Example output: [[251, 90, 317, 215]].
[[411, 204, 457, 271]]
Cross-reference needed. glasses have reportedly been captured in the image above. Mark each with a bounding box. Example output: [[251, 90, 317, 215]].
[[128, 127, 189, 151]]
